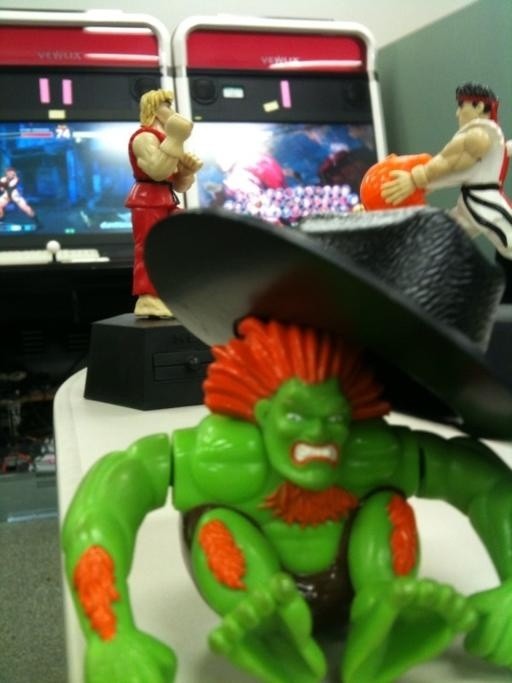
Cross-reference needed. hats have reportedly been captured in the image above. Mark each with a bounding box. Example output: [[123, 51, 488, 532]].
[[144, 204, 511, 441]]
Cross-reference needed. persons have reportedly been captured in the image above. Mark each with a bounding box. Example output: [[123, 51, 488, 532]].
[[356, 83, 510, 257], [125, 88, 202, 314], [59, 203, 511, 682]]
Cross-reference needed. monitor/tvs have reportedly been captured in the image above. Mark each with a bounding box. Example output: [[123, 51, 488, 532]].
[[190, 121, 377, 228], [0, 121, 143, 246]]
